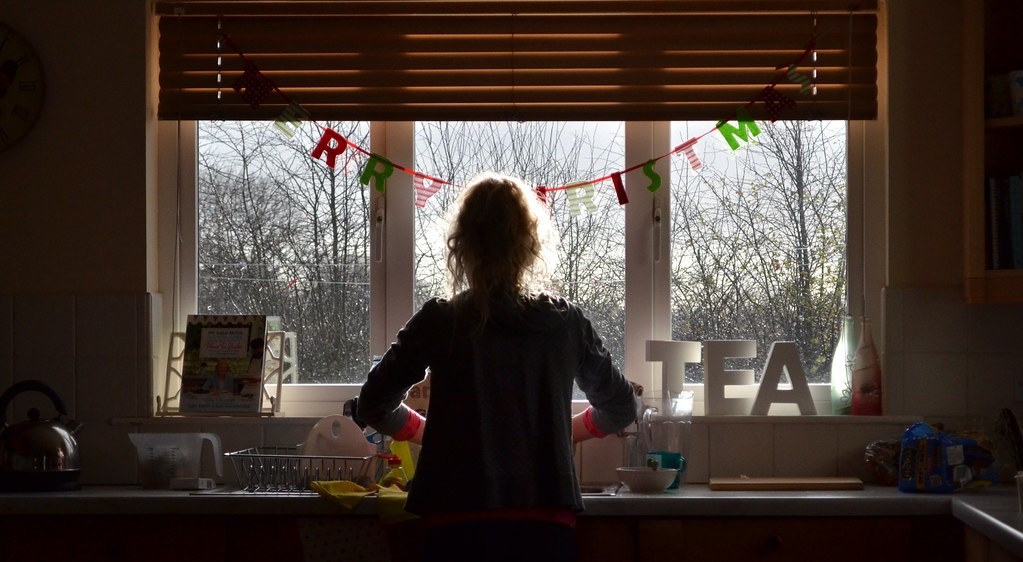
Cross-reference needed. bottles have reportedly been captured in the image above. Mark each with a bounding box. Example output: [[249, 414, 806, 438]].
[[830, 317, 858, 414], [851, 318, 881, 416]]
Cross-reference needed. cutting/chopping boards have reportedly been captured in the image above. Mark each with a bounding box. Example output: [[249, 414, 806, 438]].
[[707, 476, 863, 491]]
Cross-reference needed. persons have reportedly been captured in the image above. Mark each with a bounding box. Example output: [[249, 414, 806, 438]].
[[201, 360, 238, 393], [357, 178, 636, 562], [239, 337, 263, 399]]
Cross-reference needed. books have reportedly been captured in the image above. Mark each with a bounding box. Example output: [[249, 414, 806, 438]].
[[180, 315, 267, 413]]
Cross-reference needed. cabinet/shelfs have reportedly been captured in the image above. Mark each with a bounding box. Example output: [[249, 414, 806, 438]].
[[1, 516, 1022, 562], [959, 0, 1023, 307]]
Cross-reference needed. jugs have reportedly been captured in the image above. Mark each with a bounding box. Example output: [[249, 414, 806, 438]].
[[641, 388, 695, 484], [127, 433, 222, 490]]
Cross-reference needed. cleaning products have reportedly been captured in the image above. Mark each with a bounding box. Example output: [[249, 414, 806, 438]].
[[377, 454, 408, 490]]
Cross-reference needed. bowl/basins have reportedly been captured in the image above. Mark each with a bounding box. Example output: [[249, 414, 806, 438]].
[[616, 466, 677, 494]]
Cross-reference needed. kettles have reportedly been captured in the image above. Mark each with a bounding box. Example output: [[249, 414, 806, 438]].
[[0, 380, 87, 495]]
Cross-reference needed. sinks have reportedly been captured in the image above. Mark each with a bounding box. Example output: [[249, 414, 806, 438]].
[[576, 480, 624, 496]]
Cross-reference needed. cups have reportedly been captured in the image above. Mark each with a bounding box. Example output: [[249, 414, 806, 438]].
[[621, 431, 639, 469], [646, 451, 688, 489]]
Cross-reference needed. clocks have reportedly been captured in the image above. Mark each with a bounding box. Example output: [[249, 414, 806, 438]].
[[0, 23, 44, 151]]
